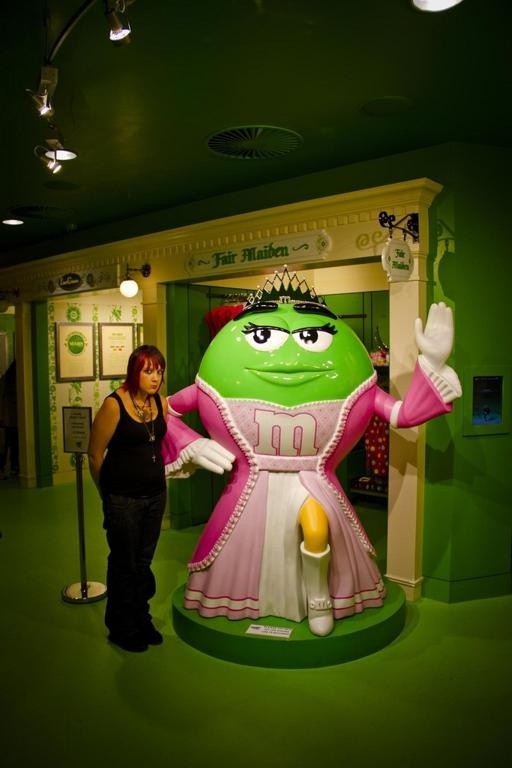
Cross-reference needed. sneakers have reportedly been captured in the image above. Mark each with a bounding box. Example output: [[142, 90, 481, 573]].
[[108, 627, 163, 653]]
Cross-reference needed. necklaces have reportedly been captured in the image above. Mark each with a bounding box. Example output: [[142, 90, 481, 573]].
[[129, 391, 156, 445]]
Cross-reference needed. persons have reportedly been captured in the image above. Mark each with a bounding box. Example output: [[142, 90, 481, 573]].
[[87, 345, 172, 653]]
